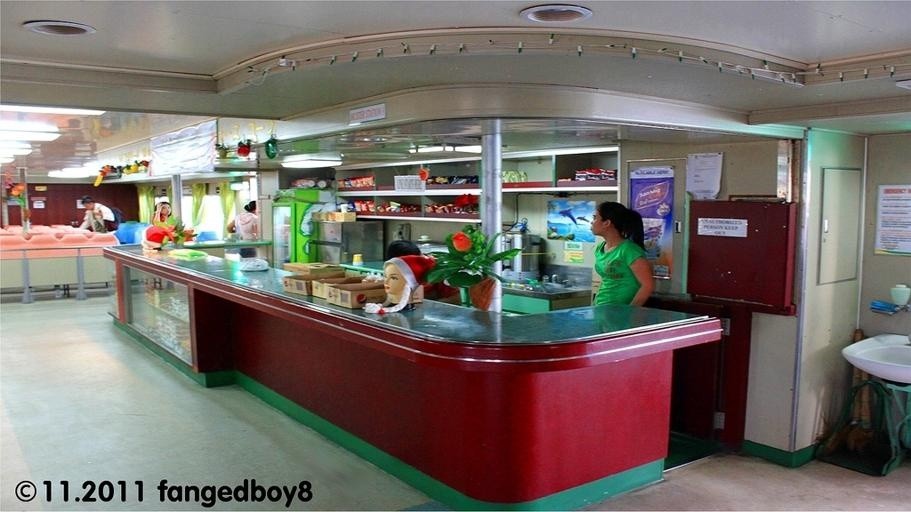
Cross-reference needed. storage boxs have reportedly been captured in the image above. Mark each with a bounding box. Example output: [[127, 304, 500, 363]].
[[311, 275, 384, 300], [281, 262, 347, 297], [325, 280, 426, 310]]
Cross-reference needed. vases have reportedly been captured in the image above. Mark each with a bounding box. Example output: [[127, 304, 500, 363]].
[[467, 275, 498, 311]]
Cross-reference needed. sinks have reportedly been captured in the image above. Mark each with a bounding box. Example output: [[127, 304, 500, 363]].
[[840, 332, 911, 384], [545, 282, 581, 292]]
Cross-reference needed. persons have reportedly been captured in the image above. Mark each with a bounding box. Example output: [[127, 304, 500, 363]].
[[75, 194, 119, 233], [150, 195, 173, 228], [589, 200, 656, 307], [224, 198, 259, 244], [361, 250, 438, 315]]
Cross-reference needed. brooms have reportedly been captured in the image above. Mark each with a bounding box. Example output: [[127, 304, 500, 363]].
[[812, 329, 862, 455], [847, 335, 881, 465]]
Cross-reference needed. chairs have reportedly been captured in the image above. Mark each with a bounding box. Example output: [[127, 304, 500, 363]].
[[0, 220, 223, 299]]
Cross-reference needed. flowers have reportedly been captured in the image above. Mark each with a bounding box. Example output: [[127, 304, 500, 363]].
[[4, 163, 31, 221], [421, 225, 524, 289]]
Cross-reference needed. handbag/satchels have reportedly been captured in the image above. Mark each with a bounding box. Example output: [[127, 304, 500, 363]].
[[103, 206, 123, 232]]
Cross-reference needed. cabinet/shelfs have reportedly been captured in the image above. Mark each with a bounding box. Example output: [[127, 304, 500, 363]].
[[114, 259, 230, 374], [333, 139, 620, 225], [502, 293, 593, 315], [642, 295, 754, 448]]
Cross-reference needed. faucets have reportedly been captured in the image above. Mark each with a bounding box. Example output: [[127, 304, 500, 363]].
[[562, 279, 572, 288]]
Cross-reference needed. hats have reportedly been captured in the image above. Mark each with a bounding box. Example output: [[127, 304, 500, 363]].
[[382, 255, 437, 293], [143, 223, 176, 249]]
